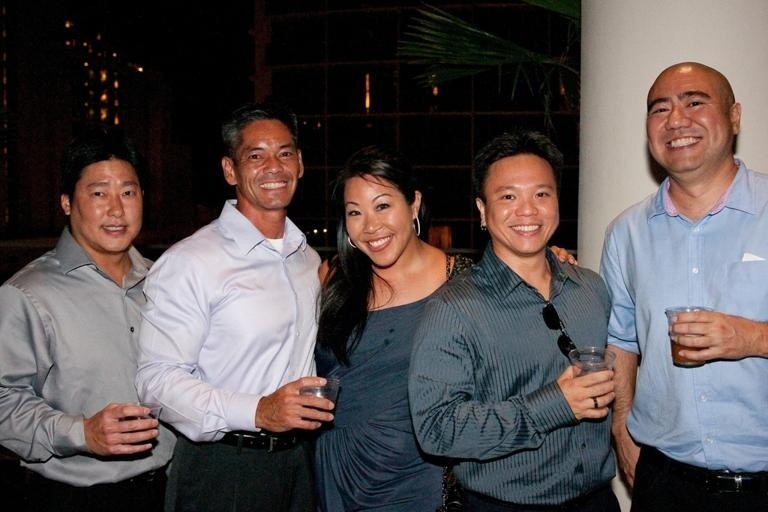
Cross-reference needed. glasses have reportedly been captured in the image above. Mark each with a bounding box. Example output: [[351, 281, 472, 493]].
[[543, 301, 579, 359]]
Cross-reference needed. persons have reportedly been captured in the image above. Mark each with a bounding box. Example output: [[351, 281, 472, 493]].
[[597, 58, 768, 512], [405, 132, 614, 511], [309, 145, 578, 512], [0, 145, 177, 509], [135, 106, 338, 512]]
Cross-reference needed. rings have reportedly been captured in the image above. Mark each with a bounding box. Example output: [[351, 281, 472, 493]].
[[592, 397, 599, 409]]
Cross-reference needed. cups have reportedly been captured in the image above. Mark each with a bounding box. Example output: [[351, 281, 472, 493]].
[[664, 306, 713, 369], [569, 348, 616, 382], [119, 402, 163, 442], [299, 377, 340, 420]]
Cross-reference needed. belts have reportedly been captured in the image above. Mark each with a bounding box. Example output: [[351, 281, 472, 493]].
[[643, 446, 767, 495], [216, 430, 301, 453], [456, 479, 611, 511], [129, 471, 156, 483]]
